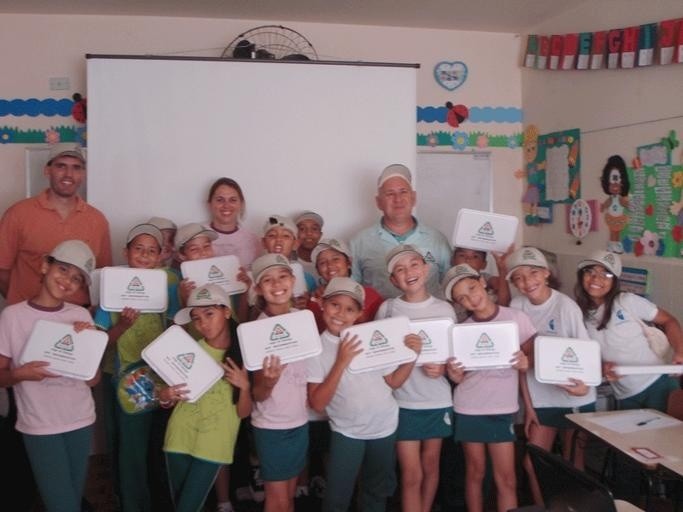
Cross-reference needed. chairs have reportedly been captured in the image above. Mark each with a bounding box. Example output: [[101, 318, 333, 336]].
[[527, 443, 645, 512]]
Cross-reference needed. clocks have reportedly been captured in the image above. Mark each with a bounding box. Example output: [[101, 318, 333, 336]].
[[568, 200, 591, 239], [435, 62, 468, 91]]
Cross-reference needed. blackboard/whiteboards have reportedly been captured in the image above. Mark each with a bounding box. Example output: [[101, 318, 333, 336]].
[[24, 147, 495, 266]]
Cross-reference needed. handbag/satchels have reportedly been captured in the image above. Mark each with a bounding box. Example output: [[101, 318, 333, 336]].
[[110, 361, 170, 415], [642, 324, 674, 363]]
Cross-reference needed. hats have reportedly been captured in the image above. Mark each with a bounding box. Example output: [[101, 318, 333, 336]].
[[49, 238, 96, 283], [441, 263, 481, 302], [46, 142, 85, 164], [126, 217, 218, 251], [577, 250, 622, 279], [387, 243, 424, 274], [308, 235, 351, 264], [264, 211, 324, 238], [252, 253, 291, 285], [504, 248, 548, 282], [377, 164, 411, 188], [323, 277, 365, 310], [174, 284, 230, 326]]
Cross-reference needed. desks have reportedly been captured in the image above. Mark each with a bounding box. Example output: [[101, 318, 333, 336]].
[[563, 408, 683, 495]]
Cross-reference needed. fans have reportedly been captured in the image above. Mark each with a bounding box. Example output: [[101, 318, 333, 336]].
[[218, 26, 319, 59]]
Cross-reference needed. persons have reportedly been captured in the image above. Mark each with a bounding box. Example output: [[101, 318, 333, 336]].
[[1, 241, 102, 511], [348, 164, 452, 300], [95, 177, 382, 512], [306, 277, 422, 512], [374, 244, 457, 511], [442, 263, 537, 511], [573, 251, 683, 498], [448, 247, 511, 323], [0, 142, 113, 416], [504, 246, 596, 506]]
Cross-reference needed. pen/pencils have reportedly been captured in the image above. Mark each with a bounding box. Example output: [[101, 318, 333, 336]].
[[638, 417, 660, 426]]
